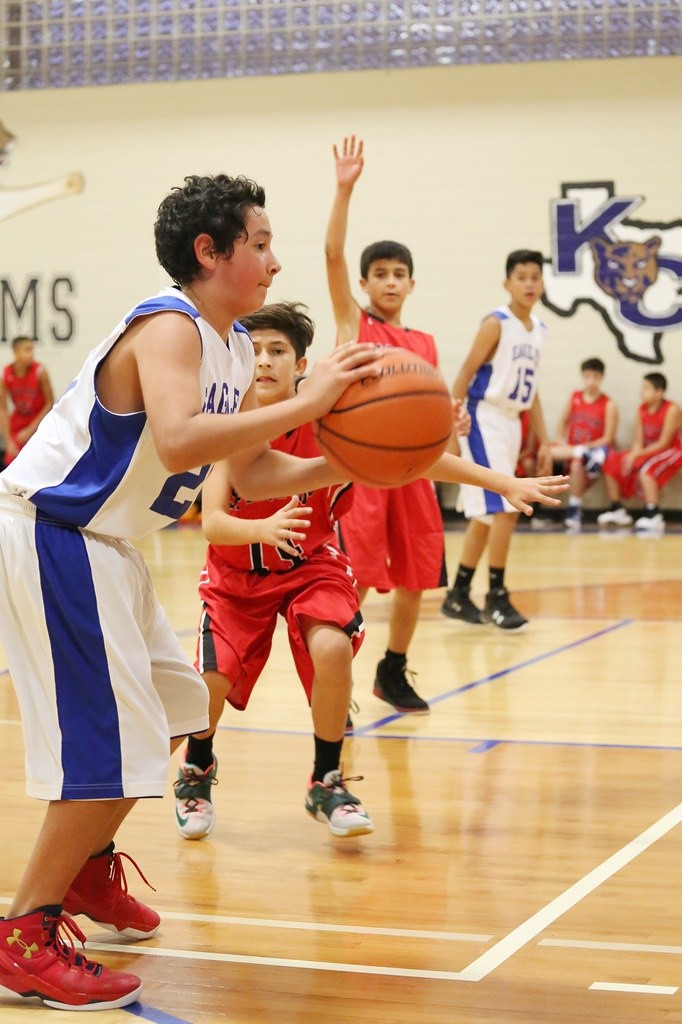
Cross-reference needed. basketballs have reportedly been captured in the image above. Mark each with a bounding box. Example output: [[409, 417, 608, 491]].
[[313, 346, 455, 488]]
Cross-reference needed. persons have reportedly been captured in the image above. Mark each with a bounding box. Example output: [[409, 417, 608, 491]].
[[174, 303, 571, 842], [0, 174, 384, 1010], [515, 357, 682, 534], [444, 249, 545, 633], [0, 335, 54, 466], [323, 136, 472, 736]]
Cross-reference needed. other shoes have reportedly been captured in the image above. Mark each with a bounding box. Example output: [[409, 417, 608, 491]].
[[633, 512, 667, 531], [562, 505, 584, 530], [530, 509, 555, 527], [597, 507, 631, 526]]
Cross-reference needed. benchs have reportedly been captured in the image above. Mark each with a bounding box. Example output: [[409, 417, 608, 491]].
[[439, 466, 682, 512]]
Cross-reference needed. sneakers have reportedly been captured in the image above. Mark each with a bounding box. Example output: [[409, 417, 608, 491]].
[[481, 588, 529, 634], [301, 760, 375, 838], [57, 845, 162, 940], [170, 745, 220, 841], [371, 661, 431, 716], [344, 697, 361, 737], [1, 904, 143, 1012], [440, 586, 486, 624]]
